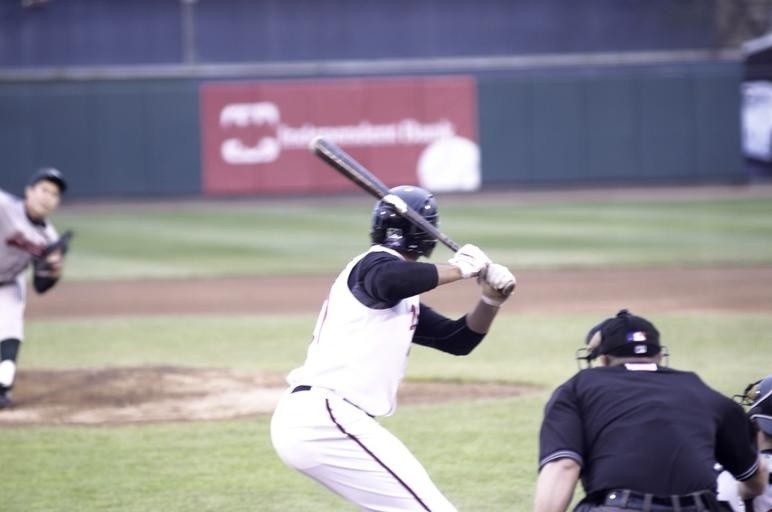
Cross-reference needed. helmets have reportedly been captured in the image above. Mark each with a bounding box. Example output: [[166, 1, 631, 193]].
[[370, 185, 439, 258], [731, 377, 772, 435], [576, 310, 669, 370]]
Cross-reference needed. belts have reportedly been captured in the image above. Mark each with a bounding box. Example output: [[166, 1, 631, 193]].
[[292, 385, 311, 393], [630, 493, 707, 506]]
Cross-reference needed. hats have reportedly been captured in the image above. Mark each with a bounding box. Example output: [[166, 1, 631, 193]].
[[29, 168, 67, 192]]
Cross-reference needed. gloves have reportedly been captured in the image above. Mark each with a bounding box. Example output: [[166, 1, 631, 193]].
[[477, 264, 516, 307], [448, 244, 492, 280]]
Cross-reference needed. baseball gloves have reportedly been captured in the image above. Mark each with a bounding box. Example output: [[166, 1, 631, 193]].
[[30, 231, 74, 292]]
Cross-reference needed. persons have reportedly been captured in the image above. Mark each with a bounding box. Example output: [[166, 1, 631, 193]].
[[270, 185, 517, 511], [0, 167, 68, 410], [533, 306, 768, 512], [740, 376, 772, 512]]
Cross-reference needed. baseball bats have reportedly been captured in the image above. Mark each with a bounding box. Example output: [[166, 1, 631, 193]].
[[312, 134, 515, 295]]
